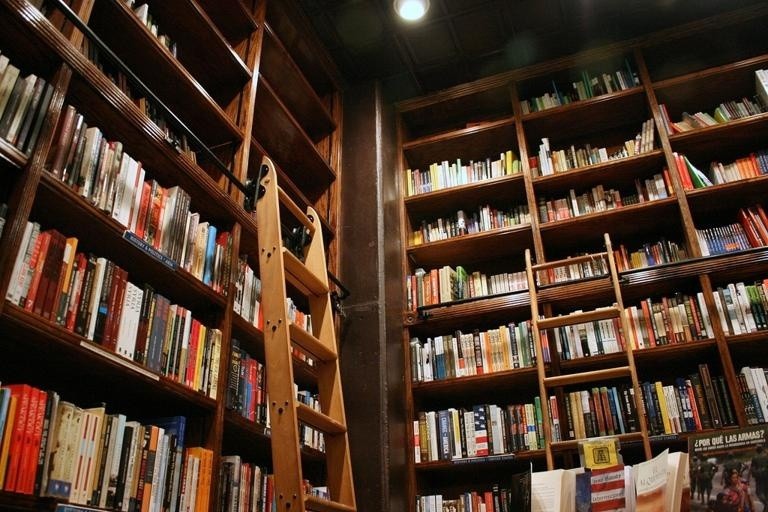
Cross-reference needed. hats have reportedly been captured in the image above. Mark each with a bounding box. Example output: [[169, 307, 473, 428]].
[[754, 444, 765, 450]]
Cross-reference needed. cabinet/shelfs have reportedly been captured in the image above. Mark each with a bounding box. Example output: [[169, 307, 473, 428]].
[[395, 4, 768, 511], [1, 1, 345, 512]]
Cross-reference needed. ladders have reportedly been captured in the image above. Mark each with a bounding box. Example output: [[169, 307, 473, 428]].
[[525, 234, 653, 471], [256, 156, 358, 512]]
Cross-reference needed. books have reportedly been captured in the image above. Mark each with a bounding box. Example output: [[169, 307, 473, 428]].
[[688, 424, 768, 512], [0, 381, 212, 512], [634, 448, 687, 512], [407, 265, 538, 313], [0, 203, 9, 238], [553, 291, 715, 361], [736, 366, 768, 425], [519, 57, 641, 115], [0, 54, 53, 157], [82, 34, 197, 164], [511, 462, 575, 512], [695, 203, 768, 257], [46, 104, 233, 297], [673, 148, 768, 191], [465, 113, 506, 129], [407, 151, 521, 196], [566, 364, 739, 439], [659, 69, 768, 136], [529, 118, 655, 178], [712, 278, 768, 334], [28, 0, 74, 32], [414, 395, 562, 464], [576, 465, 634, 512], [415, 471, 510, 512], [537, 167, 674, 224], [411, 316, 552, 382], [225, 338, 326, 452], [4, 221, 223, 401], [124, 0, 177, 58], [547, 238, 690, 284], [409, 204, 531, 246], [221, 455, 330, 512], [233, 257, 317, 370]]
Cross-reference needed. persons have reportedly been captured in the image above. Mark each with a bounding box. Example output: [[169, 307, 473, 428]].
[[746, 443, 768, 512], [696, 456, 718, 505], [690, 456, 703, 500], [712, 492, 728, 512], [722, 473, 745, 512], [730, 467, 756, 512], [723, 451, 743, 491]]
